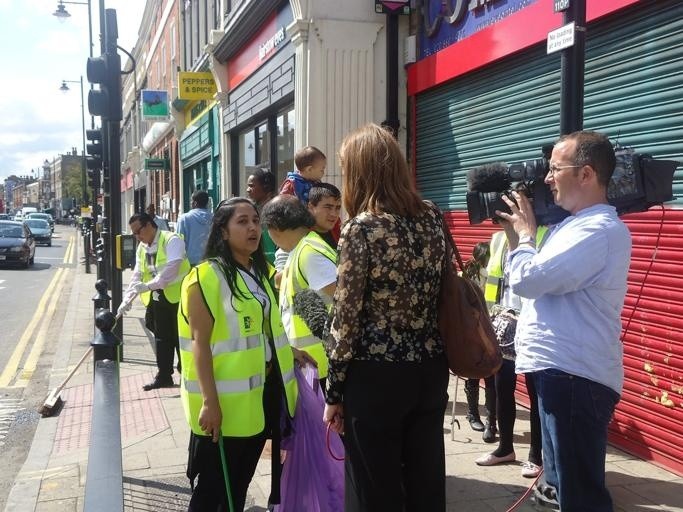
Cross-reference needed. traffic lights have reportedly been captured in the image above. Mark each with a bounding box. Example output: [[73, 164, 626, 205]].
[[84, 53, 121, 192]]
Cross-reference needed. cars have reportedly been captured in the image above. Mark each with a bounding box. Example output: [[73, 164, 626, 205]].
[[0, 220, 36, 270], [0, 206, 56, 248]]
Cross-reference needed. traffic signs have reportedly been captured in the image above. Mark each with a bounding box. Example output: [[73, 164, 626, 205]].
[[545, 20, 577, 56]]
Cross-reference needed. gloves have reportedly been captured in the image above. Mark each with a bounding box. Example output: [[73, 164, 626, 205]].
[[131, 281, 151, 294], [117, 296, 133, 315]]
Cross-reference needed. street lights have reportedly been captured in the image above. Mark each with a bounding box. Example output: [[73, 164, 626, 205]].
[[49, 0, 97, 131], [57, 74, 89, 209], [30, 167, 41, 209]]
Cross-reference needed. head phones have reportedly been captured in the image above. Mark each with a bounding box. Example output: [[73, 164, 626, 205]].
[[261, 167, 271, 191]]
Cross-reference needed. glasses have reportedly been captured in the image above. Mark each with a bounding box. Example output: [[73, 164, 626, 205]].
[[548, 161, 583, 171], [132, 223, 145, 234]]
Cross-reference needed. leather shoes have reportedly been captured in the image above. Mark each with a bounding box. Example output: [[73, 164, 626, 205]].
[[466, 410, 497, 443], [142, 373, 173, 390], [476, 449, 515, 466], [522, 457, 543, 478]]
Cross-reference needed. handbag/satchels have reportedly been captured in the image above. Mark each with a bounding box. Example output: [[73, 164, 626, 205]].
[[427, 199, 503, 380], [488, 304, 541, 360]]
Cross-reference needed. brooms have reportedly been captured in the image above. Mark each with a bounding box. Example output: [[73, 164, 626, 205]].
[[37, 293, 141, 417]]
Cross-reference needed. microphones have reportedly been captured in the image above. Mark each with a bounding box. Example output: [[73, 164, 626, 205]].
[[292, 288, 330, 339]]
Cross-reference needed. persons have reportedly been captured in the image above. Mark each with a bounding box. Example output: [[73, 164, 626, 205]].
[[470, 222, 555, 479], [115, 146, 350, 512], [459, 242, 500, 444], [318, 119, 455, 511], [492, 127, 632, 512]]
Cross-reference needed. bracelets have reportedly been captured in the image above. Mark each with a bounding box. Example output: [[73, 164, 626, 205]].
[[517, 235, 535, 247]]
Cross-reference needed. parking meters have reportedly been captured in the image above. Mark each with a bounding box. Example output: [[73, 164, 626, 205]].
[[107, 232, 139, 346]]
